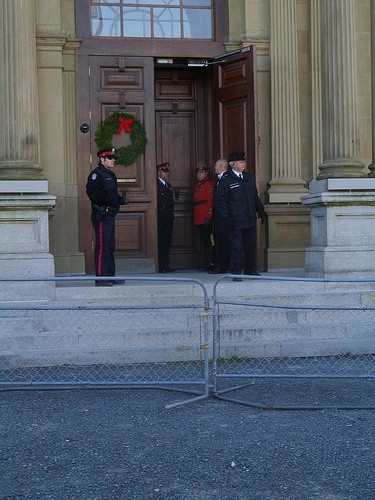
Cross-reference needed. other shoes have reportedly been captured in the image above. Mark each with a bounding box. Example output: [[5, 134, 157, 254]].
[[159, 268, 176, 273], [199, 265, 210, 272], [208, 269, 227, 274], [95, 279, 125, 286], [243, 273, 262, 276], [233, 277, 242, 281]]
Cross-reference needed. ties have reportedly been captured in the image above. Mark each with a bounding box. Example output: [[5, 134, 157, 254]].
[[239, 174, 242, 179], [165, 182, 169, 190]]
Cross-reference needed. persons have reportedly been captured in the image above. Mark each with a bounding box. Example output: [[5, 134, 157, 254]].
[[214, 150, 265, 281], [208, 159, 230, 274], [86, 147, 129, 286], [156, 162, 176, 273], [192, 166, 215, 272]]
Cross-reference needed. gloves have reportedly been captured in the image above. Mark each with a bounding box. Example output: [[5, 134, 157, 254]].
[[222, 215, 232, 233], [256, 207, 266, 224]]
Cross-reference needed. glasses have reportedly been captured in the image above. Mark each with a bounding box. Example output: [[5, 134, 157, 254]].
[[106, 157, 115, 160]]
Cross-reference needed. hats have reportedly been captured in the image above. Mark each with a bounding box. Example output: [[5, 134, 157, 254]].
[[155, 161, 169, 172], [97, 146, 117, 161], [194, 161, 209, 171], [227, 150, 247, 162]]
